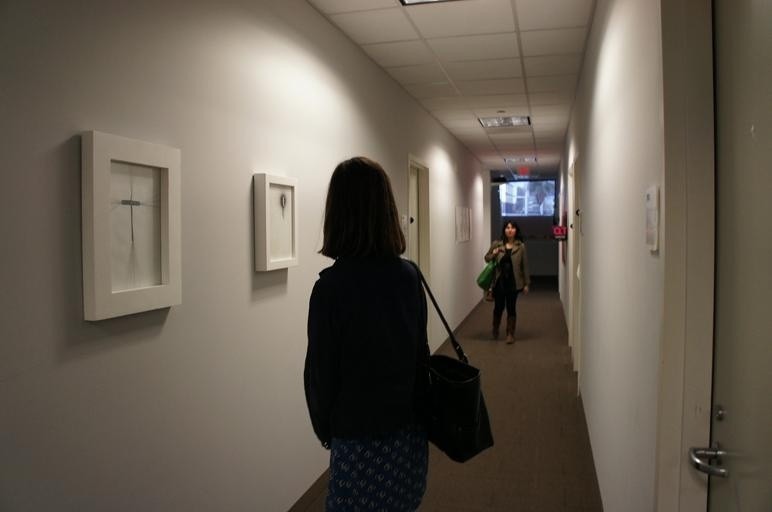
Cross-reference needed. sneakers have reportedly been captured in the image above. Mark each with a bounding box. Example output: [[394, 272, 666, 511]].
[[493, 326, 515, 344]]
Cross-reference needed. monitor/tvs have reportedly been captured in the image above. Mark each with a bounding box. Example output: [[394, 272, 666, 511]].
[[498, 178, 556, 218]]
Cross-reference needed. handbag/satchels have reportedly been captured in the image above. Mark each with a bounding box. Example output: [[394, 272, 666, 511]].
[[477, 261, 495, 291], [425, 353, 495, 463]]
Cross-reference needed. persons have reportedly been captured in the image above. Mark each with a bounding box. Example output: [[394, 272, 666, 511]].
[[485, 220, 530, 344], [304, 156, 429, 512]]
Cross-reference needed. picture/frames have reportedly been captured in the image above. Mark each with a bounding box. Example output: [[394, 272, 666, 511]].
[[254, 172, 300, 273], [80, 128, 184, 322]]
